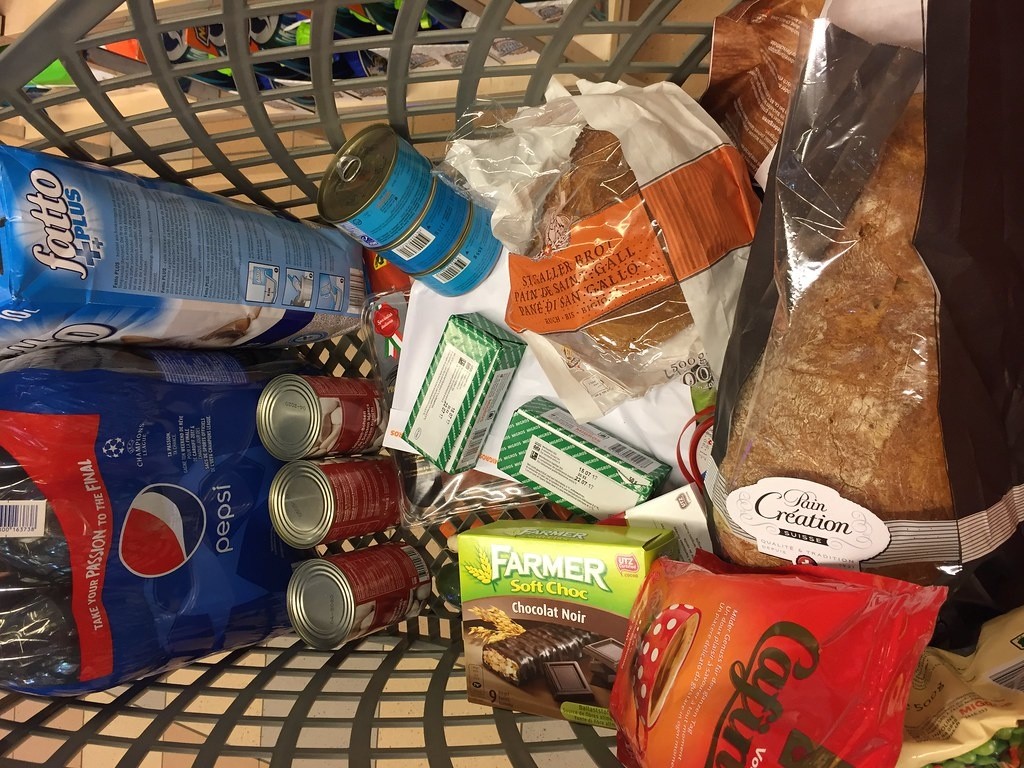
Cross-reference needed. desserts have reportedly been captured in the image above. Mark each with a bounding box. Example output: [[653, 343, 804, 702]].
[[480, 624, 624, 706]]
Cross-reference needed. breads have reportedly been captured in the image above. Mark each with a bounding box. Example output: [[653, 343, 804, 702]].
[[520, 90, 973, 606]]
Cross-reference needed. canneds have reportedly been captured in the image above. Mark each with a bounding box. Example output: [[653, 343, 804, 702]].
[[317, 123, 505, 298], [255, 373, 433, 651]]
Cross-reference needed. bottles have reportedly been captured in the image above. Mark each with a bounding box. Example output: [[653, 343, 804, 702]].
[[0, 344, 330, 702]]
